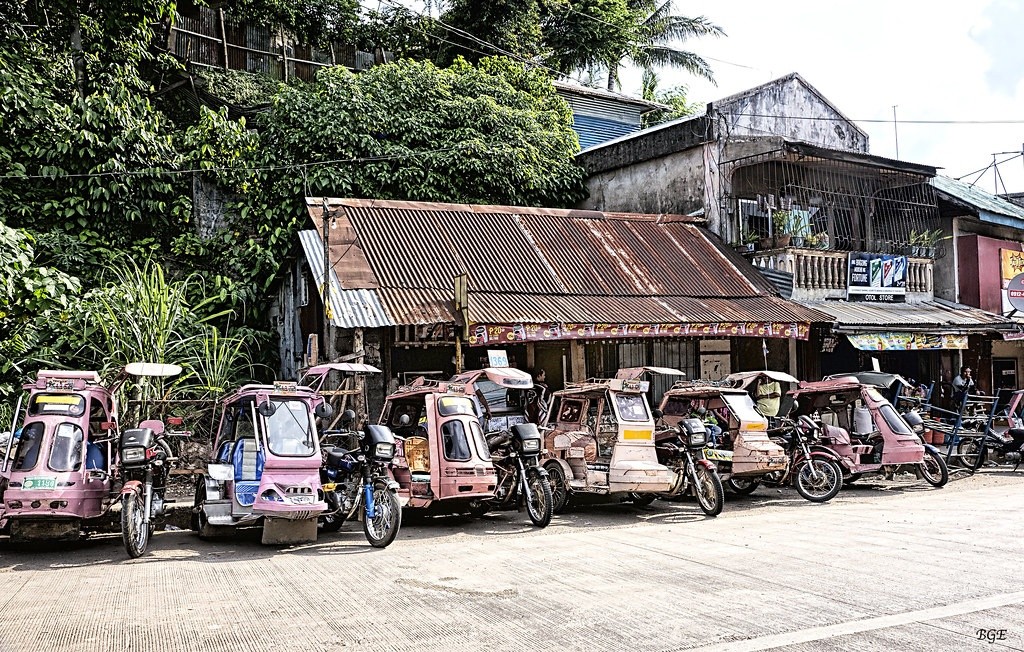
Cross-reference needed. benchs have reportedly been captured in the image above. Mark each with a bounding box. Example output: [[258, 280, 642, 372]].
[[546, 430, 597, 463]]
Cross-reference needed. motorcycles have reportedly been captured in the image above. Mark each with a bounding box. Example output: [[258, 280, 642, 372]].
[[728, 401, 843, 502], [843, 397, 948, 486], [468, 422, 559, 528], [627, 407, 724, 516], [320, 409, 409, 548], [92, 417, 191, 558]]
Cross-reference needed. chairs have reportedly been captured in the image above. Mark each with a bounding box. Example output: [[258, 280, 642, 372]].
[[393, 436, 431, 487], [215, 434, 295, 497], [823, 424, 861, 464], [22, 436, 104, 469]]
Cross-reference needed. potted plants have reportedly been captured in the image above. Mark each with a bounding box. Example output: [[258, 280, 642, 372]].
[[906, 228, 954, 258], [732, 208, 815, 254]]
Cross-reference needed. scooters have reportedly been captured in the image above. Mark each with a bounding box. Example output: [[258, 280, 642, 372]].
[[957, 404, 1024, 472]]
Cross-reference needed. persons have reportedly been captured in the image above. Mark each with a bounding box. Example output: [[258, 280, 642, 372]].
[[523, 365, 560, 427], [950, 365, 975, 421], [754, 373, 781, 429]]
